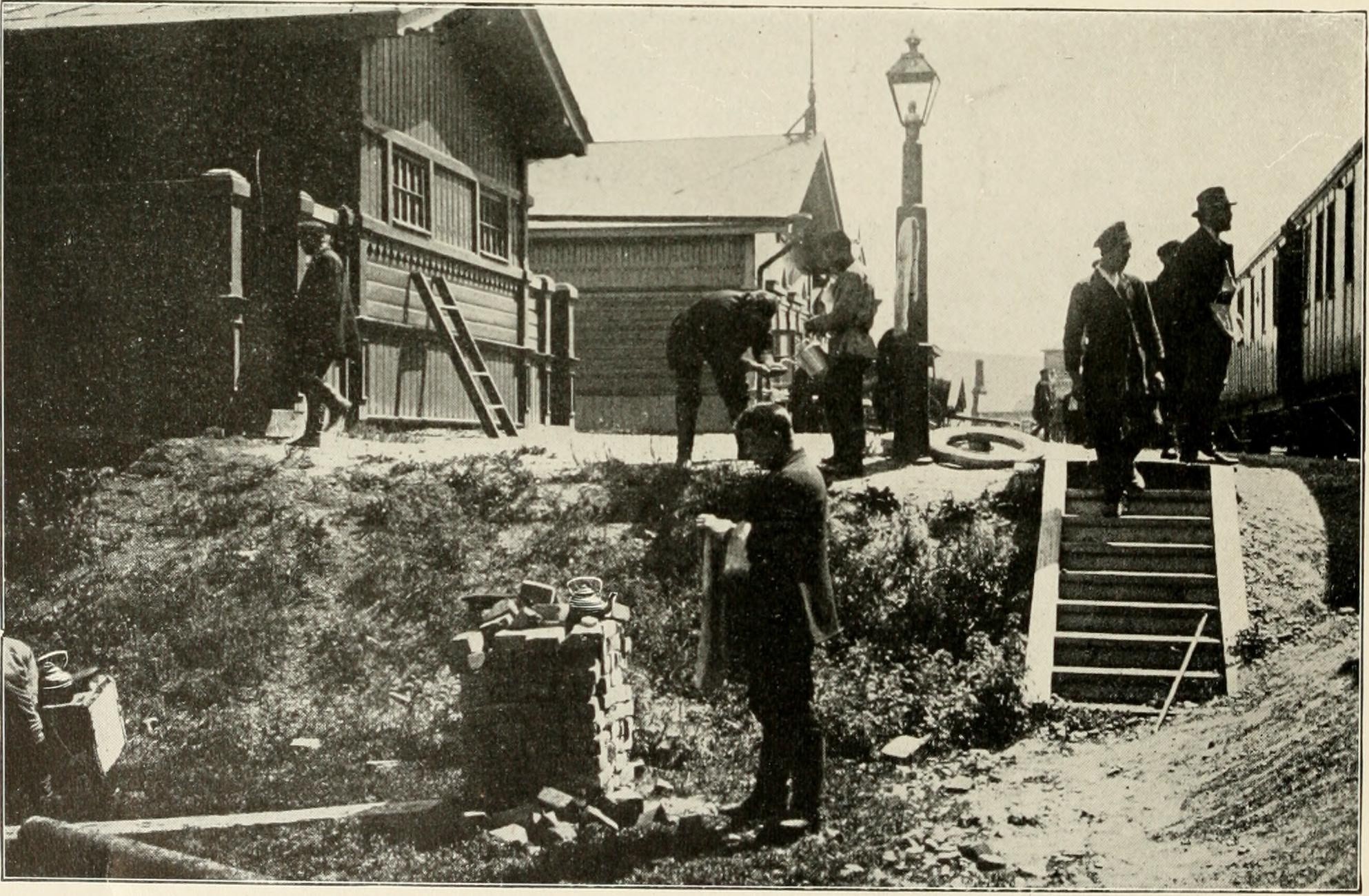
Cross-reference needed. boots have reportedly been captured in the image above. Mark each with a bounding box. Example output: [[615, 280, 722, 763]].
[[761, 788, 823, 831], [305, 376, 353, 432], [287, 393, 328, 447], [720, 776, 789, 820]]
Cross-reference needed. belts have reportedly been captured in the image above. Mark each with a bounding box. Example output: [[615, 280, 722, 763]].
[[1211, 294, 1231, 304]]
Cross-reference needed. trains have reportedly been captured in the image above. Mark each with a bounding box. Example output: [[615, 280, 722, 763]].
[[1209, 136, 1361, 462]]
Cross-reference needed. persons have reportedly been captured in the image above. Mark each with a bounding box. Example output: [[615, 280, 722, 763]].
[[666, 290, 782, 467], [275, 217, 362, 447], [804, 228, 881, 480], [1157, 186, 1241, 464], [871, 327, 899, 421], [1063, 220, 1165, 520], [1150, 240, 1185, 300], [1032, 368, 1093, 449], [695, 403, 845, 834], [0, 639, 60, 775]]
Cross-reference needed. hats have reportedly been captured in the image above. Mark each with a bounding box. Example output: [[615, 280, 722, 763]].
[[1191, 185, 1237, 216], [1156, 241, 1182, 256], [292, 220, 329, 239], [1093, 220, 1128, 254]]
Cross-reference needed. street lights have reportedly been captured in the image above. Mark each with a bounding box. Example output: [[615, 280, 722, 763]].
[[884, 25, 943, 458]]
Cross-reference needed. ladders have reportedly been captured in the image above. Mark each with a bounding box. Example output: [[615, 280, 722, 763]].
[[404, 272, 518, 438]]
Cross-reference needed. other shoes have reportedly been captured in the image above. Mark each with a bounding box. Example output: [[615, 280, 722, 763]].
[[817, 462, 862, 476], [1123, 459, 1145, 493], [822, 454, 836, 463], [1161, 452, 1177, 459], [1179, 449, 1215, 462], [1215, 452, 1240, 465], [1098, 484, 1123, 517]]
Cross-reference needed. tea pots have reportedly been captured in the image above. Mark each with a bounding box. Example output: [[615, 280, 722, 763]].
[[564, 574, 618, 626], [35, 649, 74, 707], [780, 333, 831, 376]]
